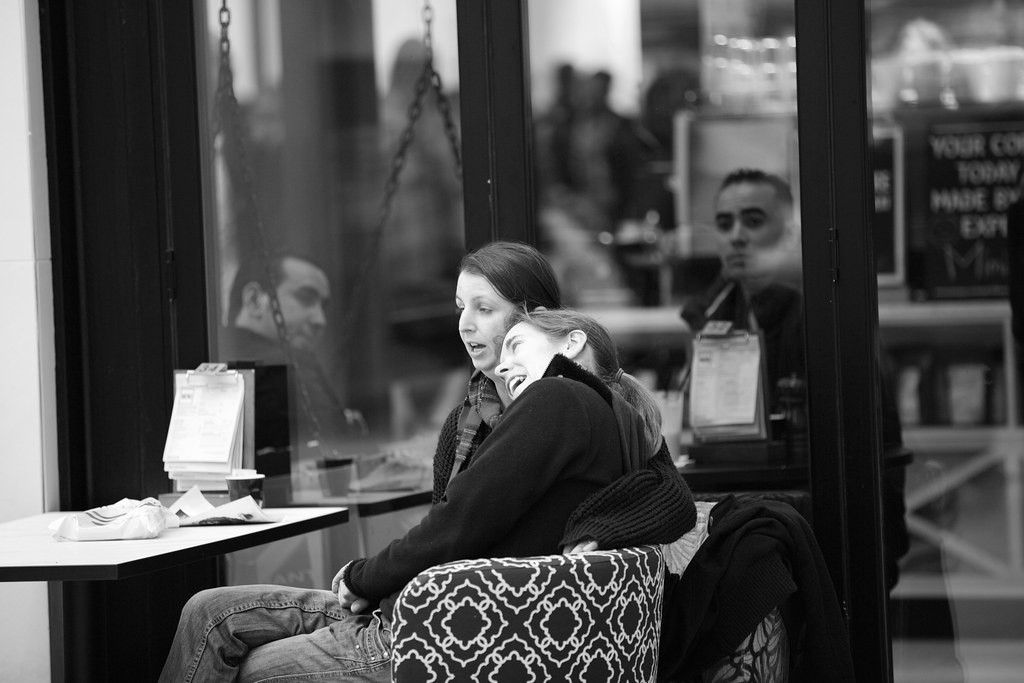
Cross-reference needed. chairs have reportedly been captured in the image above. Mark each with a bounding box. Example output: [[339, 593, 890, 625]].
[[705, 496, 792, 683], [392, 543, 666, 683]]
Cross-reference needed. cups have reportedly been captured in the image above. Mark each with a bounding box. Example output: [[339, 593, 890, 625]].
[[226, 474, 266, 507], [356, 452, 386, 481], [317, 464, 352, 498], [653, 390, 684, 463], [871, 46, 1024, 109]]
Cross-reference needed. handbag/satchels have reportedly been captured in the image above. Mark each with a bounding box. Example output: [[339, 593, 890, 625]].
[[50, 497, 164, 540]]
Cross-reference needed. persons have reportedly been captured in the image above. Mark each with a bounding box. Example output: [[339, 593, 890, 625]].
[[661, 169, 908, 594], [220, 245, 367, 495], [153, 309, 664, 683], [227, 31, 721, 301], [431, 241, 699, 554]]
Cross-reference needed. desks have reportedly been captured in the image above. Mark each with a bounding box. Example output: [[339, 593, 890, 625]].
[[269, 489, 436, 590], [0, 507, 349, 683]]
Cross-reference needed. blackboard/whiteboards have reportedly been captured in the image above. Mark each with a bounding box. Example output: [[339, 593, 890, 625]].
[[900, 106, 1020, 297]]
